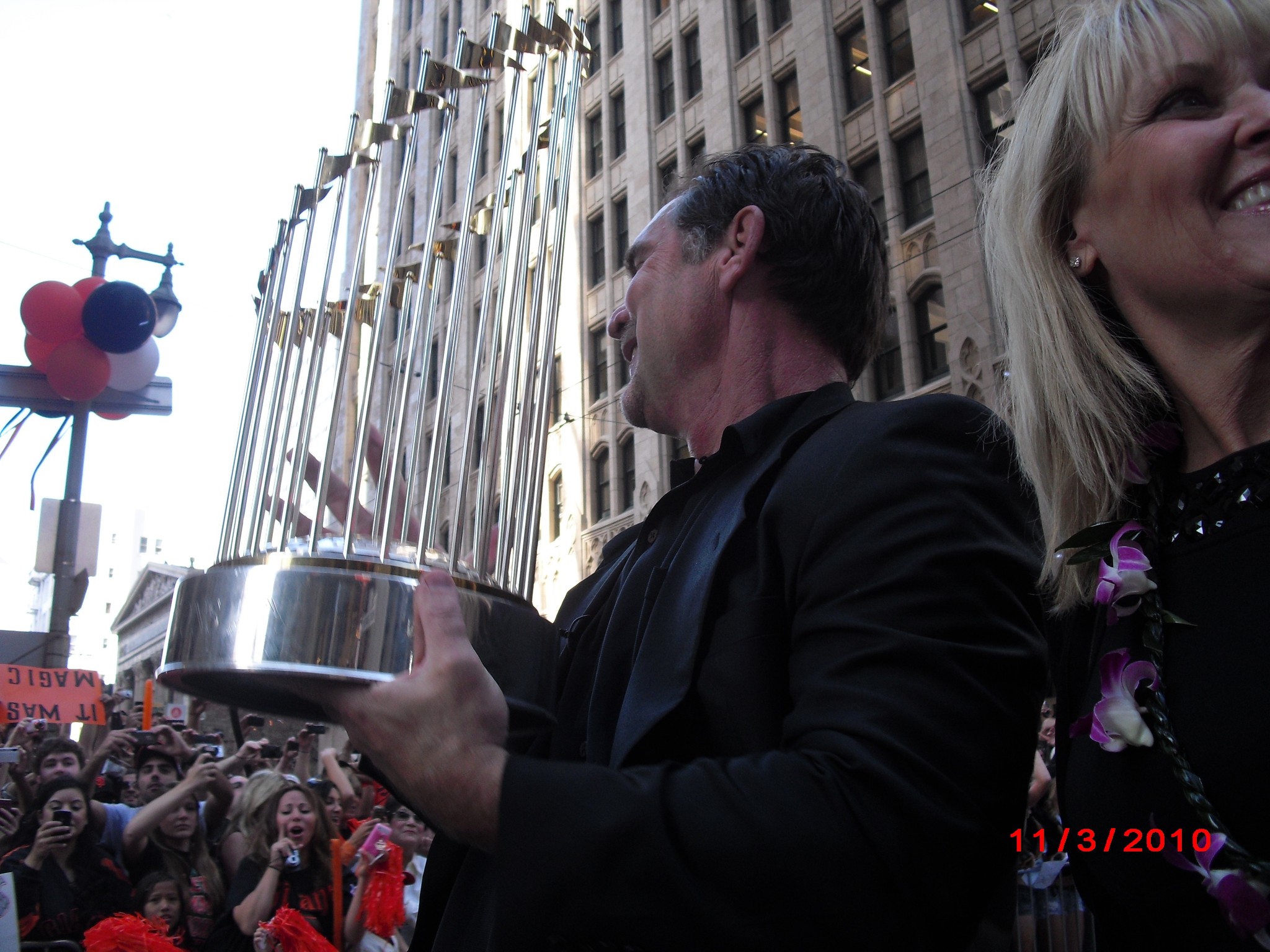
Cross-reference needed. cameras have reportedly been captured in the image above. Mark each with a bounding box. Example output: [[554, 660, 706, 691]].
[[258, 744, 282, 759], [118, 688, 133, 699], [26, 718, 48, 735], [279, 845, 302, 873]]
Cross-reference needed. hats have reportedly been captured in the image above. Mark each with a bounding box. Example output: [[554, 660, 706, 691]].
[[136, 741, 177, 765]]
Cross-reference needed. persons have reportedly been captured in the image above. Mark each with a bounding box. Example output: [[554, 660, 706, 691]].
[[259, 142, 1047, 952], [979, 0, 1270, 949], [0, 680, 1100, 951]]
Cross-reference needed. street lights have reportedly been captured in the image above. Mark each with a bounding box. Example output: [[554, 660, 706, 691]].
[[47, 198, 187, 669]]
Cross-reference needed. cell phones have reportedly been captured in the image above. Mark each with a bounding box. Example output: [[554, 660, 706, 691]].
[[307, 723, 326, 735], [247, 716, 265, 728], [110, 710, 121, 730], [0, 798, 14, 826], [286, 741, 300, 753], [50, 809, 71, 847], [191, 736, 215, 745], [129, 730, 155, 747], [203, 745, 218, 770], [174, 724, 186, 733], [135, 701, 143, 712], [360, 823, 392, 859], [372, 804, 385, 820], [0, 747, 20, 764]]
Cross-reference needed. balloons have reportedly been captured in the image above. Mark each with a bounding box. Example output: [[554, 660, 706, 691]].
[[19, 276, 162, 421]]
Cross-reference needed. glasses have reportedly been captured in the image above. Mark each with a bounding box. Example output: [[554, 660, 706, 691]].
[[120, 780, 138, 790], [165, 782, 181, 790], [390, 811, 423, 824], [306, 777, 323, 785], [230, 782, 242, 789]]
